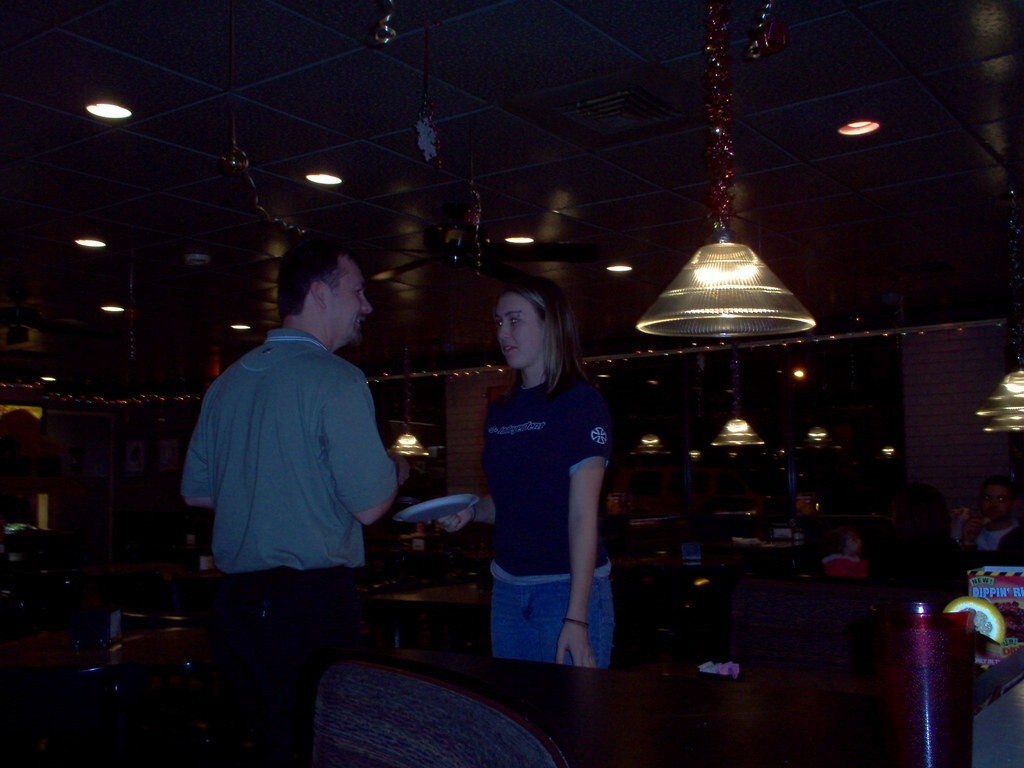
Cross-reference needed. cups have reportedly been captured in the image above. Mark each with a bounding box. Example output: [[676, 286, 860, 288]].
[[883, 603, 973, 768]]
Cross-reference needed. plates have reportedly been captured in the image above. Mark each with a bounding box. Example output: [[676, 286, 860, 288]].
[[393, 494, 480, 523]]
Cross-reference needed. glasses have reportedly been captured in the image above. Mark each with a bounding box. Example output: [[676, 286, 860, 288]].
[[981, 494, 1009, 503]]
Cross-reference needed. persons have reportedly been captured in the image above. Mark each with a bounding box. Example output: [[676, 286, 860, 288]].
[[179, 230, 414, 768], [808, 472, 1024, 595], [425, 275, 616, 768]]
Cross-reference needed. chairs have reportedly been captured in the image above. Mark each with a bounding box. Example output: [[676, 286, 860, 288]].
[[312, 662, 569, 768], [730, 583, 886, 674]]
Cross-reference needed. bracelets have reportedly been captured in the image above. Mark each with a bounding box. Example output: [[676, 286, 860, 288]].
[[562, 617, 589, 629]]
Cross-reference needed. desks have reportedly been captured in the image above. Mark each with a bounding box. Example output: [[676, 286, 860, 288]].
[[364, 584, 490, 670], [0, 628, 215, 767], [449, 655, 897, 768], [725, 537, 818, 581]]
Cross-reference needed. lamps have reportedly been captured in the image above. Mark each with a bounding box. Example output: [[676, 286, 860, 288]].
[[975, 187, 1024, 431], [634, 0, 816, 340], [712, 342, 763, 445], [385, 349, 429, 457]]
